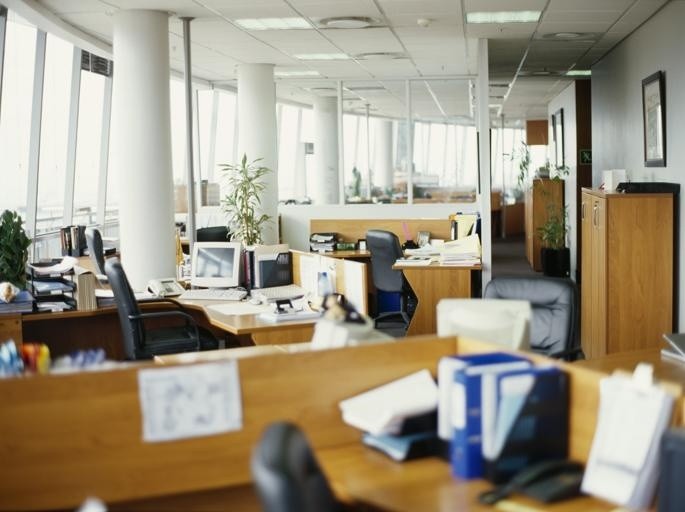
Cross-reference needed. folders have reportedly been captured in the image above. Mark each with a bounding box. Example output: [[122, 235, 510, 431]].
[[61, 225, 75, 256], [451, 361, 542, 481], [71, 225, 86, 256]]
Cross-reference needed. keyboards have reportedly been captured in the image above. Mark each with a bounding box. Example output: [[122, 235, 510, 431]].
[[178, 288, 248, 302]]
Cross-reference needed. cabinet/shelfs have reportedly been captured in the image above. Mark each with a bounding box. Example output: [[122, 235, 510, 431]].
[[525, 118, 549, 147], [580, 186, 671, 357], [523, 179, 567, 268]]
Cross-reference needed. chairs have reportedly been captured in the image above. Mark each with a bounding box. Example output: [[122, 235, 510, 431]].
[[106, 257, 218, 351], [86, 228, 112, 278], [367, 227, 412, 330], [252, 419, 340, 508], [483, 277, 581, 362]]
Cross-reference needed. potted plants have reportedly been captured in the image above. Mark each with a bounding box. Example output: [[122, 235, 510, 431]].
[[508, 146, 572, 277]]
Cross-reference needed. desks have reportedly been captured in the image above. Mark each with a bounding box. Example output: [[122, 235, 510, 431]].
[[0, 275, 318, 345], [309, 442, 656, 512], [309, 244, 491, 334]]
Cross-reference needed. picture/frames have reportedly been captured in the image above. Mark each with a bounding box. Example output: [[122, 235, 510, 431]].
[[641, 71, 670, 165], [552, 108, 569, 170]]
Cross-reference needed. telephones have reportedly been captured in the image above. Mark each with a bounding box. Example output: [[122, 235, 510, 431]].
[[146, 277, 185, 297], [475, 456, 585, 505]]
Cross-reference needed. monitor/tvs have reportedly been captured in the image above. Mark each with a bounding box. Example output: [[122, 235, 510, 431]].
[[190, 241, 245, 288]]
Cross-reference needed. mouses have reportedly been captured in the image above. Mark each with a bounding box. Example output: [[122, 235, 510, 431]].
[[248, 298, 264, 306]]
